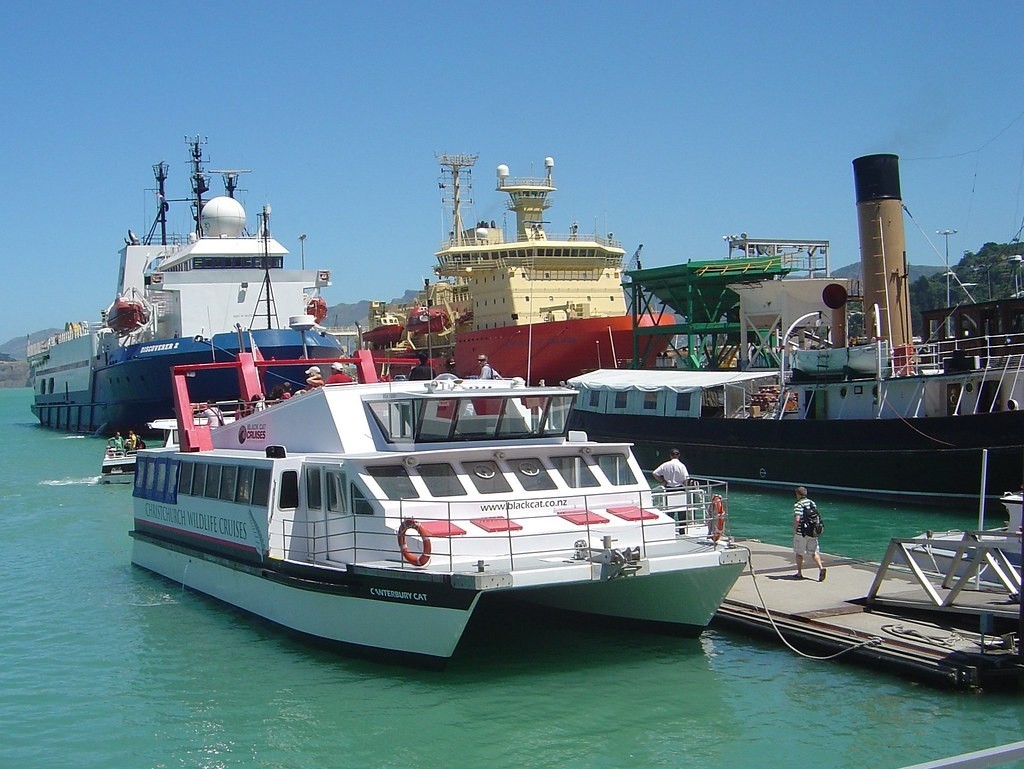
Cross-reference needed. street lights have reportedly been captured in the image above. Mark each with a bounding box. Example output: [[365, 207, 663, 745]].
[[936, 229, 959, 336]]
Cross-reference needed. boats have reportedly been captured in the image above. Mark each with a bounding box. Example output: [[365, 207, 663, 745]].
[[27, 131, 355, 435], [107, 298, 145, 330], [563, 156, 1024, 509], [129, 321, 748, 672], [362, 324, 404, 344], [893, 491, 1024, 595], [406, 306, 447, 333], [307, 296, 327, 325], [340, 152, 678, 422], [102, 439, 136, 484]]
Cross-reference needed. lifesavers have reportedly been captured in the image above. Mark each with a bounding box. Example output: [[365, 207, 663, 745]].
[[707, 495, 725, 541], [398, 520, 430, 565], [152, 274, 162, 283]]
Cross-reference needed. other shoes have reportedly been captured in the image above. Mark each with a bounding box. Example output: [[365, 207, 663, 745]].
[[792, 574, 803, 579], [819, 568, 826, 582]]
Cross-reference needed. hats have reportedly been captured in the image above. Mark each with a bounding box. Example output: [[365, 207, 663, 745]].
[[332, 362, 344, 373], [671, 448, 680, 455], [304, 365, 321, 374], [280, 382, 292, 393], [477, 354, 486, 360]]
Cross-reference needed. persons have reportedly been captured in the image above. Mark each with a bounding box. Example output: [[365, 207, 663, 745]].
[[65, 322, 82, 332], [791, 486, 826, 581], [292, 366, 324, 399], [751, 386, 798, 412], [475, 354, 493, 379], [652, 448, 690, 535], [107, 430, 146, 452], [435, 357, 462, 380], [848, 335, 877, 347], [408, 353, 437, 380], [193, 398, 224, 432], [235, 382, 291, 420], [324, 362, 354, 384]]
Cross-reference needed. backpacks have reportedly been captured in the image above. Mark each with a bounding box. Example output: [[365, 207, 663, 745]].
[[797, 501, 824, 538]]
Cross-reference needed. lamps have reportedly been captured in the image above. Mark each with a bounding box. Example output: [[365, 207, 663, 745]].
[[403, 455, 420, 467], [241, 282, 248, 288], [424, 381, 438, 394], [494, 451, 508, 459], [723, 232, 746, 241], [579, 447, 594, 454]]
